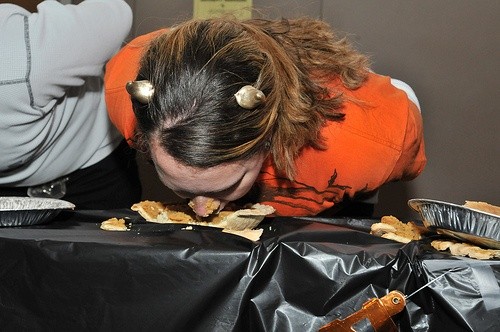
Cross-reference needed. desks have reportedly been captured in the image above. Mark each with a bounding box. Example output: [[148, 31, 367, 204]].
[[0, 209, 500, 332]]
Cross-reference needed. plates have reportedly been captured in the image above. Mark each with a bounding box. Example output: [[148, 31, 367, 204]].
[[0, 196, 75, 227], [408, 198, 500, 242], [131, 201, 274, 230]]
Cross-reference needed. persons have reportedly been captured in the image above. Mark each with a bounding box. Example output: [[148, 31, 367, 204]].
[[1, 1, 143, 209], [103, 15, 428, 220]]
